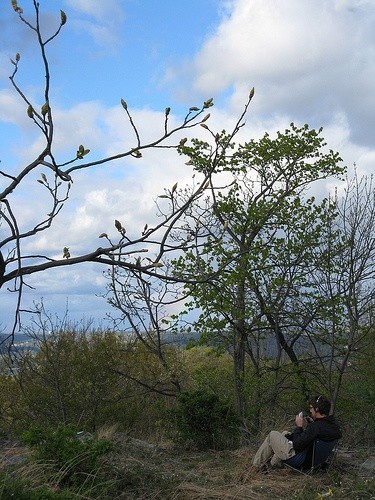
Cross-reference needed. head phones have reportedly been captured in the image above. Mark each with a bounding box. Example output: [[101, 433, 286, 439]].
[[315, 395, 323, 412]]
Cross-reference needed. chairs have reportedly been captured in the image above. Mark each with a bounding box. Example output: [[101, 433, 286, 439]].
[[259, 438, 339, 478]]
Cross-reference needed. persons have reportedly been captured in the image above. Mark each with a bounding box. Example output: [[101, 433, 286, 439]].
[[253, 395, 343, 474]]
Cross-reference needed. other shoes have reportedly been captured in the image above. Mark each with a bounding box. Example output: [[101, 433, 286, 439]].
[[267, 462, 284, 470], [245, 463, 268, 475]]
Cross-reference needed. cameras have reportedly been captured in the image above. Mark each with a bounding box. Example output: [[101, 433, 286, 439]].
[[303, 416, 310, 423]]
